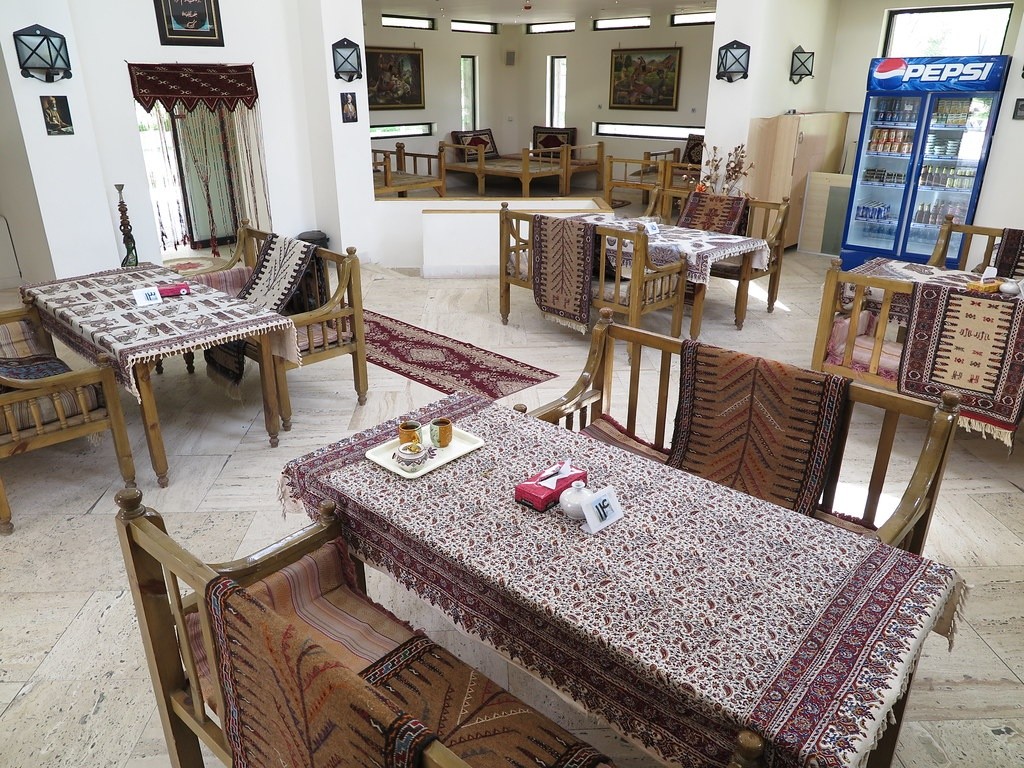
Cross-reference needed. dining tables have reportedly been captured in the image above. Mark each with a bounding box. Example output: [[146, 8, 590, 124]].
[[18, 261, 292, 487], [279, 387, 969, 768], [835, 254, 1023, 325], [556, 211, 765, 340]]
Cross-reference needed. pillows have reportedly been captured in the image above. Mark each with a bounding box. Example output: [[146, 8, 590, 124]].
[[450, 128, 499, 162], [533, 125, 578, 161], [678, 133, 704, 171]]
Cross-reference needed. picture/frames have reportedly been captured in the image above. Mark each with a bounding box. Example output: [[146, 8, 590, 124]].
[[608, 47, 683, 111], [364, 45, 426, 110]]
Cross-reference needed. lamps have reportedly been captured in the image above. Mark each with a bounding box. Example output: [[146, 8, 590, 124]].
[[789, 44, 815, 85], [13, 23, 75, 84], [332, 38, 363, 82], [715, 40, 750, 83]]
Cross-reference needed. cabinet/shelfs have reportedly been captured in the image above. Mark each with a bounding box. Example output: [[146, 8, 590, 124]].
[[753, 111, 849, 250]]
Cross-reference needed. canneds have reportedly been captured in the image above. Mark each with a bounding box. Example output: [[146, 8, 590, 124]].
[[857, 96, 988, 226]]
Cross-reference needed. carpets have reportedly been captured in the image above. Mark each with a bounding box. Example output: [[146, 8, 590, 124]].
[[163, 256, 230, 279], [325, 301, 559, 405]]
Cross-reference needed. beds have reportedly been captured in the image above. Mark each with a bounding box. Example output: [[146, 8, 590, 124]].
[[438, 137, 566, 198], [604, 147, 701, 220], [372, 142, 446, 199], [501, 140, 604, 195]]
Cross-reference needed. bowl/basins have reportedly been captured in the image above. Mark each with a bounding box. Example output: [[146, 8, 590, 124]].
[[398, 442, 429, 471]]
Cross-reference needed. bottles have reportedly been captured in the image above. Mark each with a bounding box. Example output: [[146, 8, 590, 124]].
[[914, 162, 976, 228]]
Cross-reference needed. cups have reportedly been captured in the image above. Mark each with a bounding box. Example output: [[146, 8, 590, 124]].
[[430, 418, 453, 448], [399, 420, 423, 444]]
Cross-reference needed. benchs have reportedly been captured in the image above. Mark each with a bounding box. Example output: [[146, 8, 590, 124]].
[[808, 257, 1024, 435], [113, 482, 619, 768], [927, 213, 1024, 283], [514, 307, 962, 559], [178, 218, 369, 431], [497, 201, 688, 366], [642, 182, 790, 312], [0, 295, 139, 539]]
